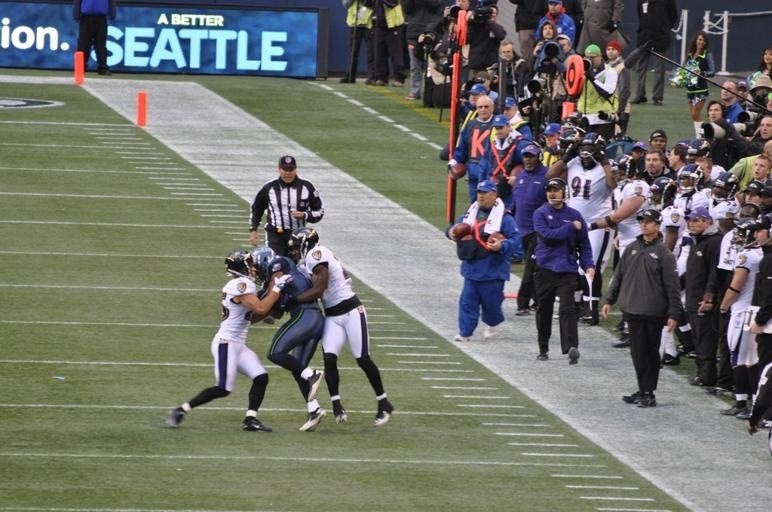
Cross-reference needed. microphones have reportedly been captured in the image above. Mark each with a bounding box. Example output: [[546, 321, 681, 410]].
[[623, 41, 650, 69]]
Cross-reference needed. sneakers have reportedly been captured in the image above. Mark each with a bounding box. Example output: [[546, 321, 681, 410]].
[[239, 415, 274, 433], [718, 402, 747, 417], [734, 408, 754, 419], [568, 347, 580, 365], [756, 418, 771, 429], [483, 325, 494, 339], [302, 407, 325, 432], [622, 391, 641, 404], [454, 334, 471, 342], [306, 369, 324, 401], [637, 394, 658, 408], [515, 303, 629, 348], [656, 344, 738, 393], [364, 76, 417, 99], [373, 397, 394, 426], [538, 353, 549, 361], [331, 400, 347, 424], [166, 406, 185, 429]]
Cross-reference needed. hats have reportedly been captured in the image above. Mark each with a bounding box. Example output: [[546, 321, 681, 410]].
[[746, 179, 762, 194], [492, 114, 507, 129], [684, 206, 712, 223], [502, 96, 517, 110], [518, 144, 541, 157], [545, 177, 566, 191], [475, 180, 498, 193], [632, 130, 707, 157], [475, 70, 490, 83], [760, 186, 771, 198], [749, 72, 771, 93], [553, 33, 621, 56], [468, 83, 486, 96], [279, 155, 298, 171], [543, 122, 560, 136], [734, 79, 748, 92], [548, 0, 561, 7], [636, 209, 663, 224]]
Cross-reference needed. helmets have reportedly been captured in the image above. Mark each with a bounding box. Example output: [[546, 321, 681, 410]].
[[224, 249, 252, 274], [710, 172, 740, 202], [578, 132, 607, 160], [677, 163, 703, 191], [647, 176, 677, 208], [731, 218, 757, 247], [287, 227, 318, 257], [252, 247, 276, 273], [753, 211, 772, 248]]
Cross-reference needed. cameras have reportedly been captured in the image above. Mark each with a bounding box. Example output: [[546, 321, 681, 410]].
[[598, 110, 626, 125], [583, 57, 593, 72]]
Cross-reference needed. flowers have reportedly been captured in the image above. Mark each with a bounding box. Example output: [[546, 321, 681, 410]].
[[669, 59, 700, 88]]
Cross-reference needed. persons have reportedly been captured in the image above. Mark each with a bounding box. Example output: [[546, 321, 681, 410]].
[[601, 209, 683, 409], [444, 180, 522, 342], [248, 156, 325, 257], [340, 1, 406, 87], [286, 228, 394, 426], [403, 1, 677, 325], [647, 32, 772, 456], [529, 179, 596, 365], [71, 0, 116, 76], [169, 249, 293, 432], [247, 246, 327, 432], [588, 153, 653, 347]]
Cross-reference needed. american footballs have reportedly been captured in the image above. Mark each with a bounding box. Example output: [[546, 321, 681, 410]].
[[450, 163, 467, 180], [511, 166, 522, 176], [453, 223, 473, 241], [488, 233, 508, 243]]
